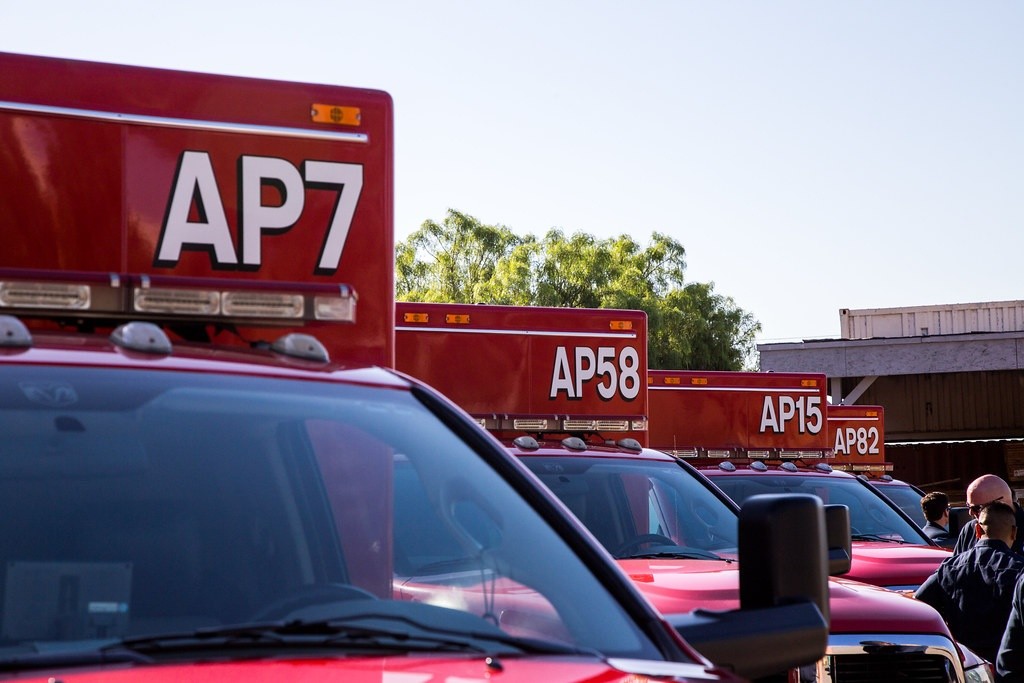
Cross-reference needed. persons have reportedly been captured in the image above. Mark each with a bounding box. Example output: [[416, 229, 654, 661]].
[[911, 474, 1024, 683]]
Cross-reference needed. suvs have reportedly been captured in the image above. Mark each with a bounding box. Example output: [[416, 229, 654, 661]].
[[387, 438, 995, 683], [0, 315, 851, 683], [685, 456, 959, 602]]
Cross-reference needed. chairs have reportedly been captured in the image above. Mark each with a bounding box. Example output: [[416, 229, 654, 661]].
[[129, 433, 307, 623]]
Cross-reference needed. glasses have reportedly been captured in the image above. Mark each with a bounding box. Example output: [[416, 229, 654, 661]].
[[944, 504, 951, 510], [969, 496, 1003, 512]]
